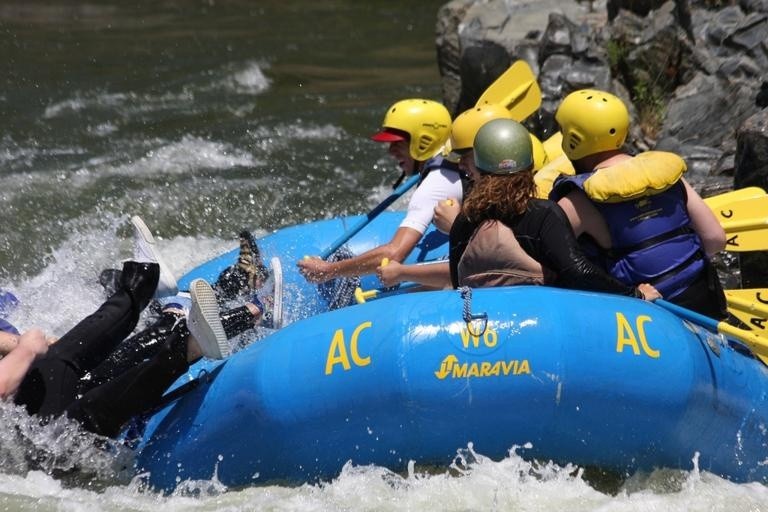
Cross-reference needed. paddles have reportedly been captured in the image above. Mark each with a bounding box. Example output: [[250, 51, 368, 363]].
[[649, 186, 767, 367], [302, 60, 542, 262]]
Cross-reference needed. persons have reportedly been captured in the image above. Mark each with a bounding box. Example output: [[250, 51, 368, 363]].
[[0, 212, 282, 488], [378, 105, 576, 289], [431, 90, 727, 318], [449, 119, 663, 302], [299, 98, 471, 284]]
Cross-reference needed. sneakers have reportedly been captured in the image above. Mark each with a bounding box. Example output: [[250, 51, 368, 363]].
[[101, 216, 229, 360], [257, 257, 283, 329], [237, 230, 269, 289]]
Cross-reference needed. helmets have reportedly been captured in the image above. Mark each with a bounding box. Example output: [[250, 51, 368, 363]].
[[450, 104, 514, 152], [473, 118, 532, 174], [555, 89, 629, 160], [371, 98, 452, 161]]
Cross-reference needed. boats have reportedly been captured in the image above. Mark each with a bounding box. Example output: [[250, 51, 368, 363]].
[[114, 210, 766, 495]]
